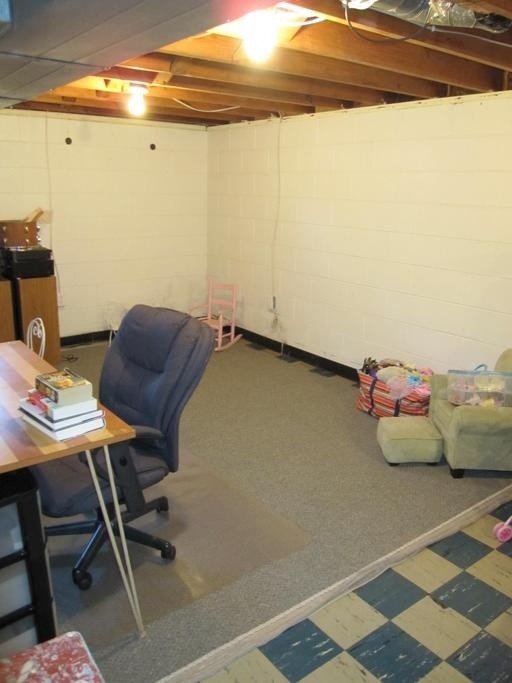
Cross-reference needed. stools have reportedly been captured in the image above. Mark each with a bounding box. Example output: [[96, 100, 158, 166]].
[[377, 416, 444, 467]]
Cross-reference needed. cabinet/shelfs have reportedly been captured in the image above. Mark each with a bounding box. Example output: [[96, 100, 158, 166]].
[[0, 275, 60, 365]]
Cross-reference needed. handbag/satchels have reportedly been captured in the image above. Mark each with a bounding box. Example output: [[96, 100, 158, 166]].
[[358, 362, 430, 420]]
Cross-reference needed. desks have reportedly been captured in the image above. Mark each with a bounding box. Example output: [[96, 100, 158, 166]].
[[0, 340, 136, 642]]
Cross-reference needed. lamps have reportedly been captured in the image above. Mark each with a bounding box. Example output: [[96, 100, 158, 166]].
[[125, 82, 149, 117]]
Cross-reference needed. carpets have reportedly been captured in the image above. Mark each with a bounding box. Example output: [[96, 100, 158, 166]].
[[157, 475, 512, 683]]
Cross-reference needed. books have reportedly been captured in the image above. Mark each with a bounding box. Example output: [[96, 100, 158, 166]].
[[16, 368, 105, 442]]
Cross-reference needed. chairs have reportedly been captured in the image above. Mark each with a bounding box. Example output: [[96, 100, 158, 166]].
[[29, 304, 214, 589], [188, 280, 242, 351], [428, 348, 512, 478]]
